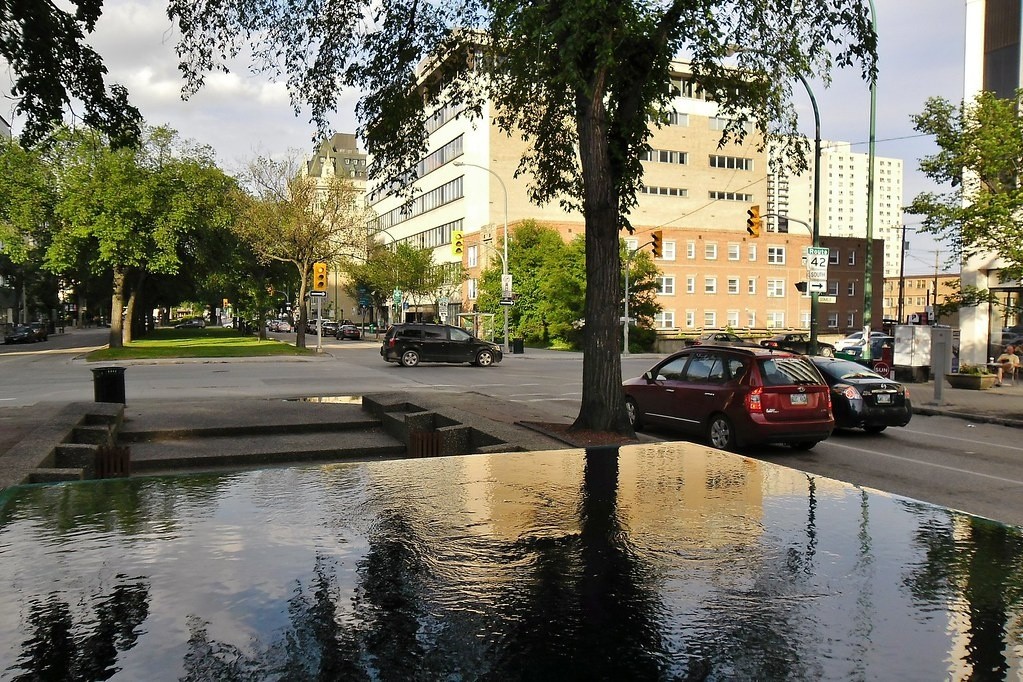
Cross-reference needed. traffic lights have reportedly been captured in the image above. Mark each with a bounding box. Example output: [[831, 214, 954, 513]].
[[268, 286, 273, 297], [452, 230, 464, 256], [652, 230, 663, 259], [313, 263, 327, 292], [746, 205, 760, 238]]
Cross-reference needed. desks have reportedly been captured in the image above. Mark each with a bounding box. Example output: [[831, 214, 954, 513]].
[[982, 363, 1023, 385]]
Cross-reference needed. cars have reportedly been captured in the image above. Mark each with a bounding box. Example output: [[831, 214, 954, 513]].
[[174, 319, 206, 330], [760, 332, 834, 357], [685, 333, 761, 349], [803, 354, 912, 435], [622, 346, 834, 451], [336, 325, 360, 340], [4, 327, 35, 344], [1002, 324, 1023, 373], [222, 319, 250, 330], [841, 337, 894, 360], [834, 332, 890, 351], [265, 318, 354, 337]]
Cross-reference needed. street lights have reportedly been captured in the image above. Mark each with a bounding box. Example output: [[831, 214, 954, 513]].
[[368, 227, 398, 323], [720, 43, 822, 356], [452, 162, 509, 354]]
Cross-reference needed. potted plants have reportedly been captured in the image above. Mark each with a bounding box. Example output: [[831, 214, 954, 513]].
[[946, 363, 998, 390]]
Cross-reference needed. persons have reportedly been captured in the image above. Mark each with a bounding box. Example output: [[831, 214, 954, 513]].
[[996, 345, 1019, 384], [96, 312, 100, 327], [85, 312, 92, 328]]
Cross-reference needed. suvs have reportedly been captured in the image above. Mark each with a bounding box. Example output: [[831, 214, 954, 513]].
[[379, 322, 503, 368], [22, 322, 48, 342]]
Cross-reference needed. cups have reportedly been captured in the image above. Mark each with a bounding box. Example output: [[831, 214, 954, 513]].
[[990, 357, 995, 363]]
